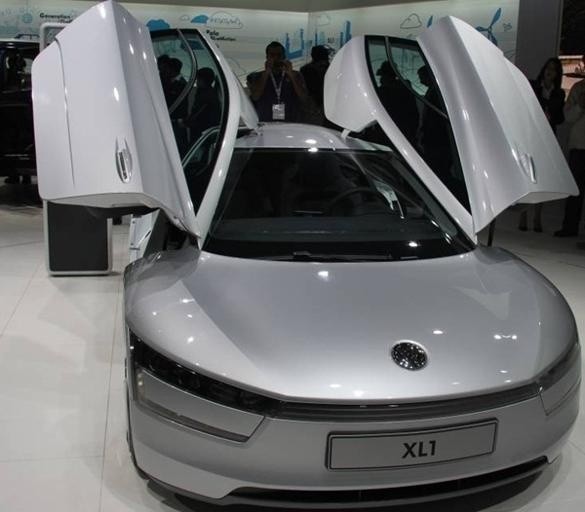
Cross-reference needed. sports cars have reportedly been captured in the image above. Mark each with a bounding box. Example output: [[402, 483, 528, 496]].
[[30, 0, 581, 512]]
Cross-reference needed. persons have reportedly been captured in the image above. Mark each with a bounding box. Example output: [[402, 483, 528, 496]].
[[177, 67, 222, 144], [156, 55, 188, 152], [517, 57, 566, 234], [375, 60, 419, 152], [2, 54, 32, 183], [417, 64, 453, 187], [299, 45, 345, 131], [554, 79, 584, 249]]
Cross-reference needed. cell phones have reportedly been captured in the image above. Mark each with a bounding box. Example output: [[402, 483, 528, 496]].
[[270, 59, 284, 72]]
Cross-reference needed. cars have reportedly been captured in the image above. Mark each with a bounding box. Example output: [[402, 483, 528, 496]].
[[0, 32, 53, 171]]
